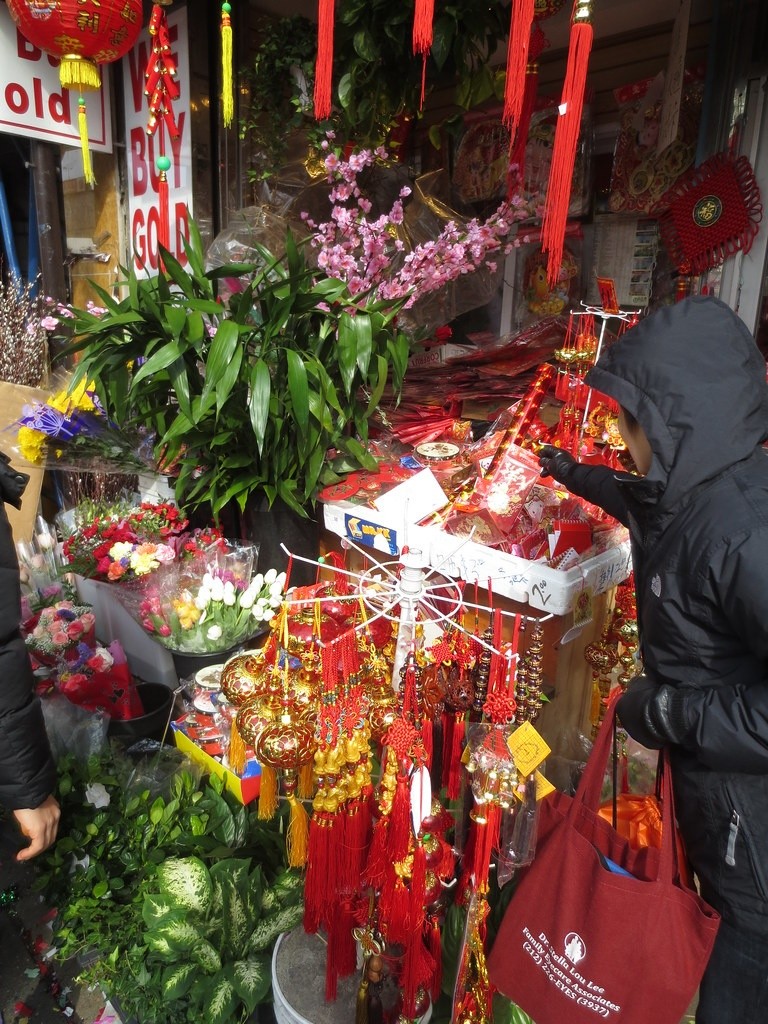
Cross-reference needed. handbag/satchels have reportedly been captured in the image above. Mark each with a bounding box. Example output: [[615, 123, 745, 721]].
[[486, 693, 722, 1024]]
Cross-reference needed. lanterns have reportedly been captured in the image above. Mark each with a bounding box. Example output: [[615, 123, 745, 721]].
[[218, 583, 638, 1024], [6, 0, 144, 189]]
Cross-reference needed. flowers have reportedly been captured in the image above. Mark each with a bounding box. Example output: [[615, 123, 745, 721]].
[[0, 270, 288, 716], [300, 128, 537, 318]]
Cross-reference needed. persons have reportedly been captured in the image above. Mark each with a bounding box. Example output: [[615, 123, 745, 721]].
[[538, 294, 768, 1024], [1, 452, 62, 862]]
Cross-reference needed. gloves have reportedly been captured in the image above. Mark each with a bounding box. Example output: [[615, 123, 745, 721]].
[[537, 445, 579, 486], [617, 676, 680, 751]]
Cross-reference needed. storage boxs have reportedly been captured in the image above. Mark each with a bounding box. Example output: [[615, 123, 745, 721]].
[[429, 531, 635, 615], [323, 500, 441, 568], [171, 712, 261, 807]]
